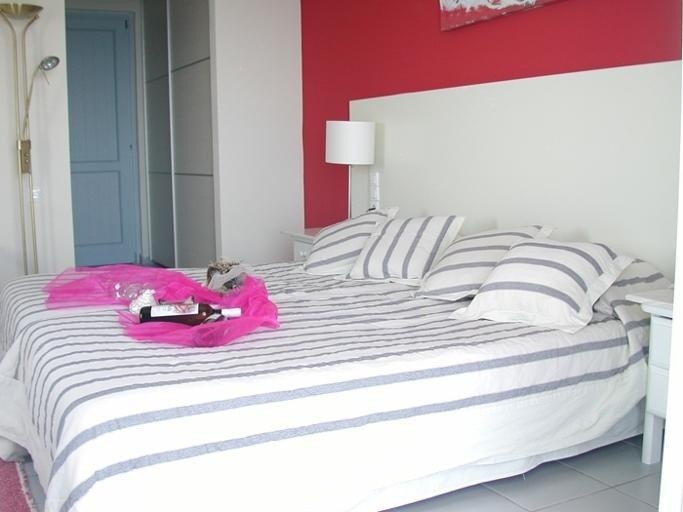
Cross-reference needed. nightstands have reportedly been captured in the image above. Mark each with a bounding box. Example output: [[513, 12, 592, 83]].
[[281, 227, 325, 262], [625, 287, 674, 464]]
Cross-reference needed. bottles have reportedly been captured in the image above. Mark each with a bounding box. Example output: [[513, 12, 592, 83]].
[[138, 301, 242, 327]]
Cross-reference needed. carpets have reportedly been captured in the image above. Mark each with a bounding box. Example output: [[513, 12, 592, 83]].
[[0, 458, 40, 512]]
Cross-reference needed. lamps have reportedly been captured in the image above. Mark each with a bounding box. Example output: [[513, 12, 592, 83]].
[[324, 120, 378, 219], [0, 2, 59, 277]]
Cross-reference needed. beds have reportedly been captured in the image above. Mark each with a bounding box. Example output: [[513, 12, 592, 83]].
[[0, 211, 667, 512]]
